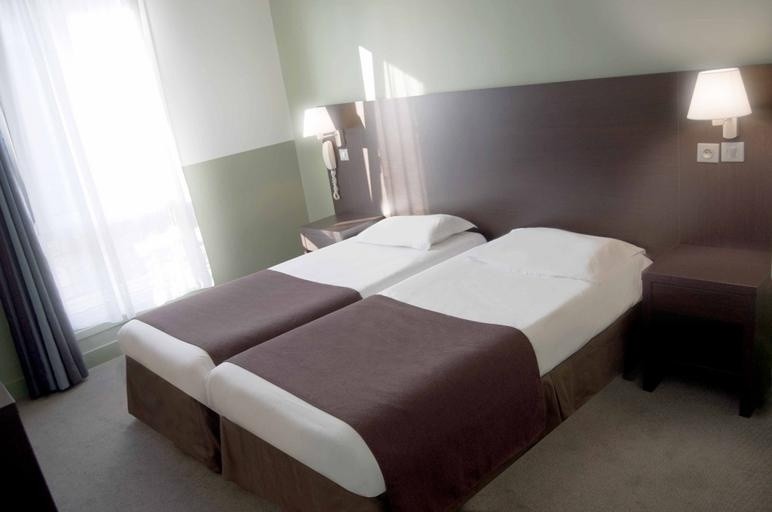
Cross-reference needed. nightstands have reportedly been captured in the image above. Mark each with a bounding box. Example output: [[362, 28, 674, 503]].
[[642, 243, 772, 418], [298, 213, 384, 252]]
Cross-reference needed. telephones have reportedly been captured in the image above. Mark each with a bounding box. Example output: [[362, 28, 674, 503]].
[[322, 141, 337, 170]]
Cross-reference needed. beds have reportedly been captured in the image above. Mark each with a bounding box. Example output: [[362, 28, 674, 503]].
[[192, 250, 654, 512], [114, 228, 488, 478]]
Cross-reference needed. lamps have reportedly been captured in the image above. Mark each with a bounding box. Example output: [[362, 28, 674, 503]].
[[686, 66, 753, 138], [301, 106, 344, 148]]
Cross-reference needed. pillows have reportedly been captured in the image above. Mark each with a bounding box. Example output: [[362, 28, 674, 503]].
[[470, 227, 645, 286], [357, 214, 478, 250]]
